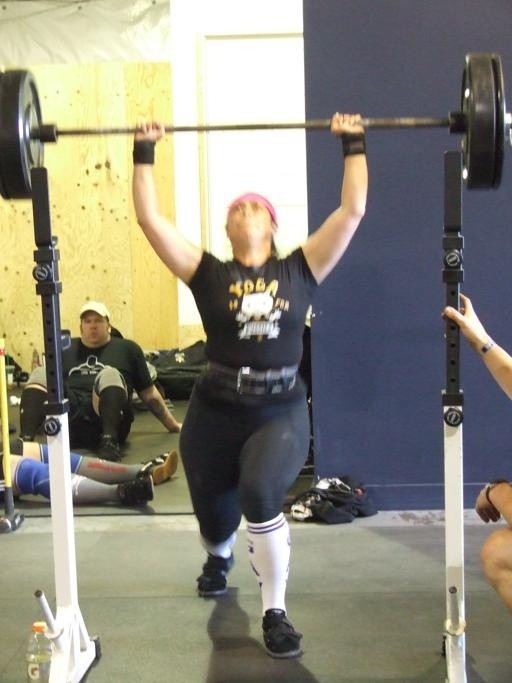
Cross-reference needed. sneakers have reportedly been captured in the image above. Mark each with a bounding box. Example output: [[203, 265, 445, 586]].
[[96, 433, 123, 463], [137, 449, 178, 485], [196, 550, 234, 596], [262, 608, 303, 658], [117, 474, 155, 506]]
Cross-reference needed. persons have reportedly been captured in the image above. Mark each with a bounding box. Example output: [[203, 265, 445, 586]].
[[0, 440, 178, 507], [132, 110, 370, 660], [19, 301, 184, 462], [439, 292, 511, 615]]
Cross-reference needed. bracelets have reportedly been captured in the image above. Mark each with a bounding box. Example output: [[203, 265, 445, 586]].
[[340, 130, 367, 158], [131, 138, 157, 166], [478, 339, 497, 355], [485, 478, 512, 506]]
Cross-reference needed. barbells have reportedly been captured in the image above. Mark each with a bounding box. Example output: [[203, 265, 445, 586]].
[[0, 52, 512, 199]]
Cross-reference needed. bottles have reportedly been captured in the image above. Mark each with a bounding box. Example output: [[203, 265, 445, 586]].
[[24, 621, 55, 683], [31, 348, 39, 372]]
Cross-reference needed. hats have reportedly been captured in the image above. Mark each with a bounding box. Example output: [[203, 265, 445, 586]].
[[79, 302, 110, 323]]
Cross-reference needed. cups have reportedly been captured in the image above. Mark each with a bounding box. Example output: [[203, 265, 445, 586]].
[[5, 365, 15, 385]]
